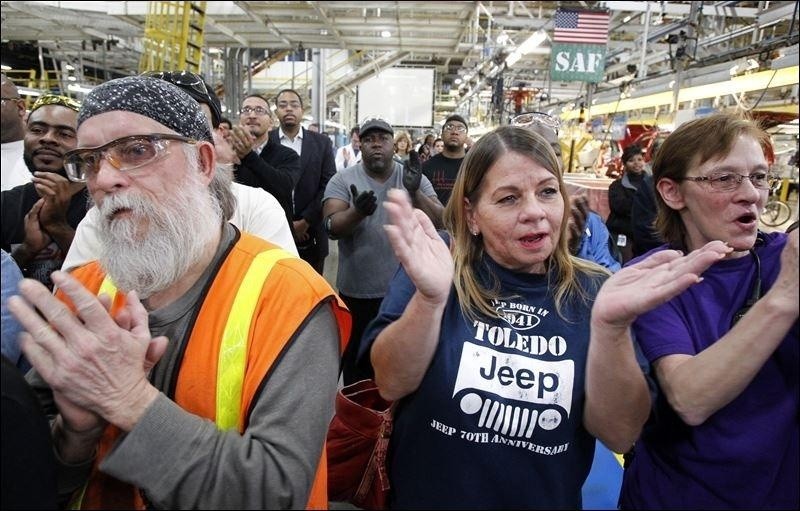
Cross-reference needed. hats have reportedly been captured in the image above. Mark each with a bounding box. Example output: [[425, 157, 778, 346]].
[[443, 116, 468, 129], [76, 77, 214, 145], [358, 115, 394, 136], [27, 94, 82, 127], [149, 73, 221, 128], [621, 147, 641, 165]]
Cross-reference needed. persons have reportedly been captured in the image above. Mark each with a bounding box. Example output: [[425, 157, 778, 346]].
[[0, 68, 800, 510]]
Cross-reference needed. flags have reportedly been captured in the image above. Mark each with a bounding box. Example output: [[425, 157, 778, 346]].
[[549, 40, 608, 84], [553, 9, 608, 43]]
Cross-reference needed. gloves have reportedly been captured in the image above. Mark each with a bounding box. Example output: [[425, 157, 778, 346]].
[[351, 183, 378, 216], [403, 149, 422, 194]]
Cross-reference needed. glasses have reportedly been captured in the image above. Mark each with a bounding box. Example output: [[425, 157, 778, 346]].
[[139, 69, 221, 115], [681, 171, 781, 192], [509, 112, 559, 136], [62, 132, 198, 182], [238, 108, 271, 118], [444, 124, 466, 133]]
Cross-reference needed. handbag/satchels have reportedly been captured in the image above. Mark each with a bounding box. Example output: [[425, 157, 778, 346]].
[[327, 379, 396, 510]]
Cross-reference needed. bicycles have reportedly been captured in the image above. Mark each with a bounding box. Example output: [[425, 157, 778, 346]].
[[760, 179, 791, 227]]
